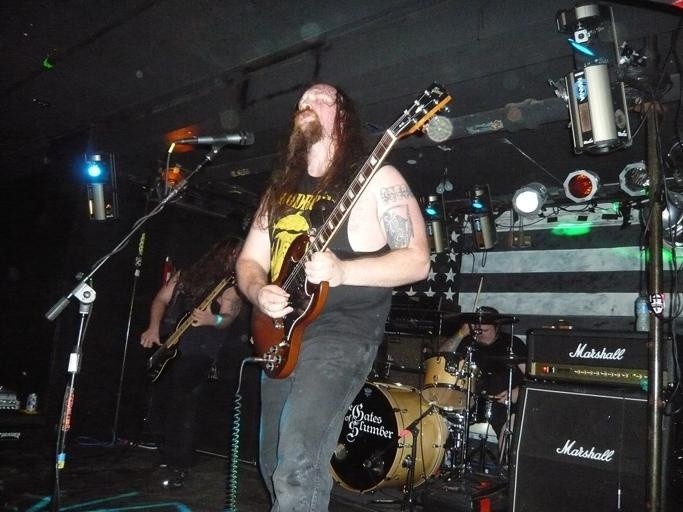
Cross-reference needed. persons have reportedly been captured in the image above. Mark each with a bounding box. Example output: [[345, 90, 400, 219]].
[[439, 304, 530, 479], [232, 80, 435, 511], [139, 232, 245, 489]]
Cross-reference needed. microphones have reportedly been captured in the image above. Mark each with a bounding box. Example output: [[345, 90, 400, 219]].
[[485, 399, 494, 420], [174, 130, 255, 147]]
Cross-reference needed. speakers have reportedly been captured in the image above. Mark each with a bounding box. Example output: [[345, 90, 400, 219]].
[[504, 380, 679, 511]]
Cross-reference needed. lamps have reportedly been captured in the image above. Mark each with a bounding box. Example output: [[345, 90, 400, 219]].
[[510, 160, 650, 246]]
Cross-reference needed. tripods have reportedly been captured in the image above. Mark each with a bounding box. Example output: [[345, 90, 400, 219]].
[[372, 426, 419, 512], [466, 420, 498, 473]]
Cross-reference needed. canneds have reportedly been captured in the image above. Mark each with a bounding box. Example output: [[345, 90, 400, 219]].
[[26, 393, 38, 412]]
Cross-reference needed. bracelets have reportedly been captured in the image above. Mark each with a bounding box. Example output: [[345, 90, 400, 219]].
[[458, 328, 465, 338], [215, 314, 223, 328]]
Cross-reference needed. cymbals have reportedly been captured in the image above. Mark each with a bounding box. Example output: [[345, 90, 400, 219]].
[[446, 312, 519, 326]]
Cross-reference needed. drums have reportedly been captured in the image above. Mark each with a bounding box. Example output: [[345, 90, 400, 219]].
[[328, 381, 449, 493], [421, 351, 477, 410]]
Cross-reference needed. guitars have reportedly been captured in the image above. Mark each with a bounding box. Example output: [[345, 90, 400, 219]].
[[145, 275, 235, 381], [249, 80, 452, 380]]
